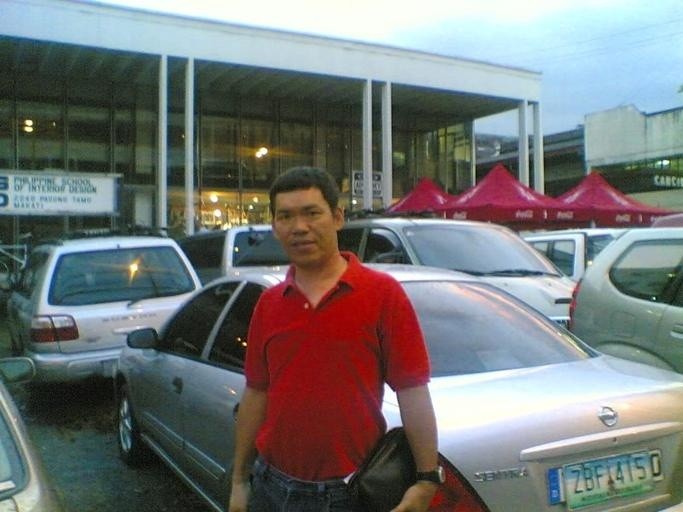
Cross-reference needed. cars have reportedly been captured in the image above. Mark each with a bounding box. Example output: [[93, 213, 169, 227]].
[[115, 262, 681, 510], [177, 224, 290, 283], [9, 225, 201, 386], [0, 356, 63, 512]]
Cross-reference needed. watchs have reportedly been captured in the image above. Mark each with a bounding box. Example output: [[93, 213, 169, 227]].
[[412, 466, 446, 484]]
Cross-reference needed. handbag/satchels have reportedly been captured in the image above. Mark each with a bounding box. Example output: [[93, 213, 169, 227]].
[[346, 426, 418, 512]]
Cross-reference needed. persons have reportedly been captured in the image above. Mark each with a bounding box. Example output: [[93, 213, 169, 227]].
[[229, 165, 446, 512]]
